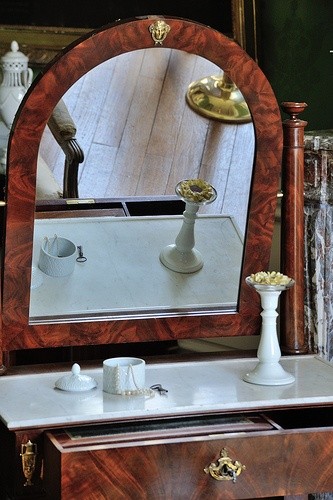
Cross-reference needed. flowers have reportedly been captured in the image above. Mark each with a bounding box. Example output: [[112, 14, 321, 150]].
[[250, 270, 292, 287]]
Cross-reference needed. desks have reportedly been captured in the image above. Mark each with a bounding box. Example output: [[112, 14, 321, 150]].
[[0, 339, 331, 500]]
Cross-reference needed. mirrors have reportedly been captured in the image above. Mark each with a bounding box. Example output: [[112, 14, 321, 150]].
[[30, 46, 255, 325]]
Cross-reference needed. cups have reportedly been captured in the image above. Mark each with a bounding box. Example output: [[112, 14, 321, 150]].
[[38, 237, 76, 277], [103, 357, 146, 394]]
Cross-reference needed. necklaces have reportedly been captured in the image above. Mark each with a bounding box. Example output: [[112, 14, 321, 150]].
[[44, 232, 60, 256], [115, 362, 154, 397]]
